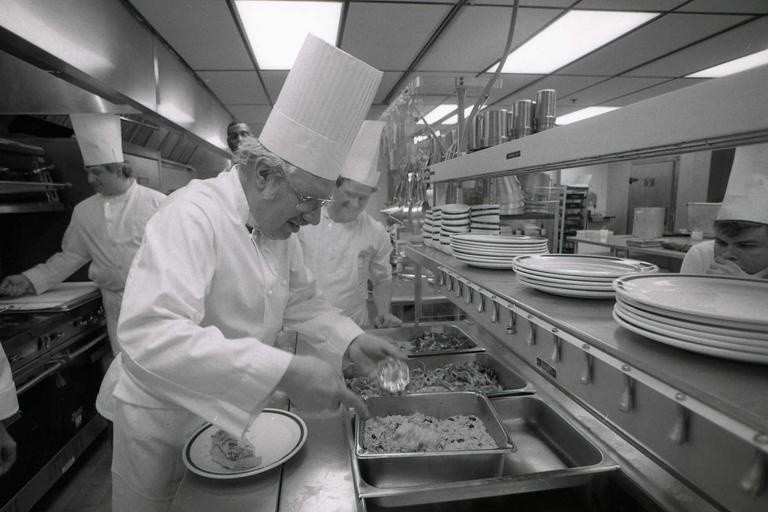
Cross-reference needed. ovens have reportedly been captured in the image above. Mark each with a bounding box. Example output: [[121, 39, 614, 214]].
[[0, 131, 110, 403]]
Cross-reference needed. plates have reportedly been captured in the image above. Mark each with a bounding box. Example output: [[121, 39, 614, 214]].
[[182, 406, 309, 481], [452, 237, 549, 267], [608, 273, 768, 364], [510, 253, 660, 302]]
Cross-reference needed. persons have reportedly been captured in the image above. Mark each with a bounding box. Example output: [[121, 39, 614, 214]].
[[679, 219, 767, 279], [0, 339, 22, 476], [279, 173, 406, 329], [90, 138, 408, 511], [1, 161, 169, 356], [226, 120, 251, 161]]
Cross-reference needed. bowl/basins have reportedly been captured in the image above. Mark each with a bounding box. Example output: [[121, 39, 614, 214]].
[[421, 204, 504, 257]]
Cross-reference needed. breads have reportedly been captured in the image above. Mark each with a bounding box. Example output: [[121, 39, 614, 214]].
[[210, 429, 262, 470]]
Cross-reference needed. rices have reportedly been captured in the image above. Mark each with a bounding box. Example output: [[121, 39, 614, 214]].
[[364, 412, 499, 454]]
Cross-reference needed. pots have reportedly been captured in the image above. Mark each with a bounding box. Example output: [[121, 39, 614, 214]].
[[378, 169, 427, 240], [481, 172, 563, 217]]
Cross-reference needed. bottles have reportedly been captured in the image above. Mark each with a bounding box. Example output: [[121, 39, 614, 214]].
[[457, 88, 558, 152]]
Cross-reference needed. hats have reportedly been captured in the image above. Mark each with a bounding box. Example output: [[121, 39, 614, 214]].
[[68, 111, 125, 167], [717, 144, 764, 224], [254, 29, 385, 186], [332, 116, 386, 191]]
